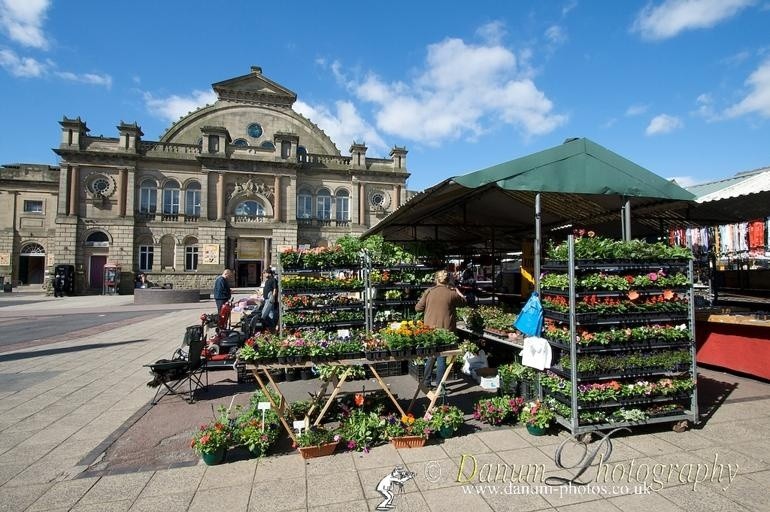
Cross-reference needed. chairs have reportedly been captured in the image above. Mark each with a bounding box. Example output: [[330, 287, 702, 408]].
[[143, 324, 210, 406]]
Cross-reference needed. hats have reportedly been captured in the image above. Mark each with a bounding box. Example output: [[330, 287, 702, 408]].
[[437, 269, 450, 284], [261, 268, 275, 273]]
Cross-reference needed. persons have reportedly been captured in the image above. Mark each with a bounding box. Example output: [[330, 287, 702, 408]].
[[453, 266, 463, 280], [414, 270, 469, 391], [136, 274, 152, 289], [259, 268, 278, 329], [53, 275, 64, 297], [214, 268, 234, 335]]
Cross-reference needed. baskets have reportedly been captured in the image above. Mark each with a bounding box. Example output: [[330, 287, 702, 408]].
[[408, 360, 454, 383], [368, 361, 401, 378], [389, 436, 426, 448], [297, 440, 339, 459], [238, 368, 255, 384]]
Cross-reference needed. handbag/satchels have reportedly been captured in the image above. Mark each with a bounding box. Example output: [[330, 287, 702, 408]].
[[519, 336, 553, 371]]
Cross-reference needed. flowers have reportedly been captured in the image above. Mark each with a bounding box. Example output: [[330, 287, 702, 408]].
[[190, 233, 697, 467]]
